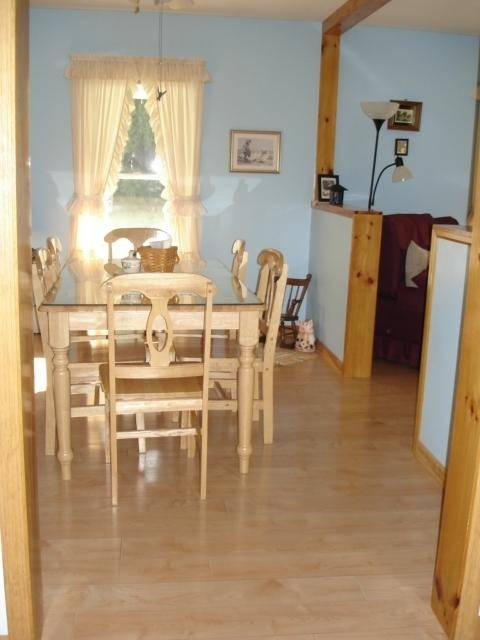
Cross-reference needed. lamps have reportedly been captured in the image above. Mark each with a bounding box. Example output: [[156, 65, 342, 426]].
[[359, 101, 415, 215]]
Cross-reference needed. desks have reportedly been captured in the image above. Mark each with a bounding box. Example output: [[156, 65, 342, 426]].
[[37, 249, 269, 482]]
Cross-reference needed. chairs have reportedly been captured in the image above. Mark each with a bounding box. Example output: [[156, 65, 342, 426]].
[[103, 229, 173, 252], [98, 272, 219, 506], [44, 236, 148, 341], [167, 239, 248, 413], [373, 212, 465, 364], [173, 247, 289, 450], [30, 247, 146, 457]]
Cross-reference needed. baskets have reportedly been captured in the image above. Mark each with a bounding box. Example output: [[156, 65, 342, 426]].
[[138, 245, 180, 272]]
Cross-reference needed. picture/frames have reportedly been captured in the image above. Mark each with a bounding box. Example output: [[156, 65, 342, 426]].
[[388, 98, 422, 132], [395, 139, 408, 155], [230, 129, 281, 173]]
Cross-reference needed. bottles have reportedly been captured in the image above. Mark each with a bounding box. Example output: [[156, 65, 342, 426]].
[[120, 250, 141, 272]]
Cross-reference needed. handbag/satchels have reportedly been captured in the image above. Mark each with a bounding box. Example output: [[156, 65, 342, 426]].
[[296, 319, 316, 353]]
